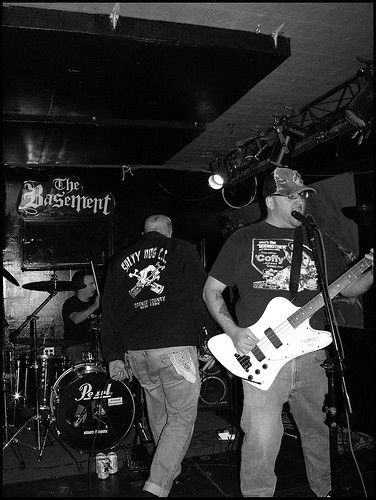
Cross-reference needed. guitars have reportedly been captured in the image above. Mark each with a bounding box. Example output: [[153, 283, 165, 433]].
[[206, 250, 375, 391]]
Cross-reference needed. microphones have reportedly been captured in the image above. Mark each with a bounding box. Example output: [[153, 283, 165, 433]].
[[291, 210, 316, 229]]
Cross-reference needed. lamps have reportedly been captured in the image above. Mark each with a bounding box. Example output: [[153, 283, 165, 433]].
[[208, 157, 241, 191], [343, 75, 374, 129], [267, 123, 307, 166]]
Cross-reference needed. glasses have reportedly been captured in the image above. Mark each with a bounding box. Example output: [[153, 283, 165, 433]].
[[288, 191, 309, 200]]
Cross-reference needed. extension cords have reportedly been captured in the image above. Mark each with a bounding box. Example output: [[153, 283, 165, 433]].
[[217, 430, 235, 440]]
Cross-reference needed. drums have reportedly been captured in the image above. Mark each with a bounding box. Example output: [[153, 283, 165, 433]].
[[50, 362, 136, 453], [14, 354, 71, 410], [0, 348, 19, 392]]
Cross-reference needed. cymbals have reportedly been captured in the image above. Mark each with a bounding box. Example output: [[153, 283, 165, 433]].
[[0, 264, 20, 287], [8, 337, 81, 347], [22, 280, 87, 292]]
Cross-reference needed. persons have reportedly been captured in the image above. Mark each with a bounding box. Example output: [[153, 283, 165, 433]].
[[62, 271, 100, 365], [202, 168, 376, 500], [100, 215, 232, 500]]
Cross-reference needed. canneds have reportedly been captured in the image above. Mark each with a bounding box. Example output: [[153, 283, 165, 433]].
[[96, 452, 117, 479]]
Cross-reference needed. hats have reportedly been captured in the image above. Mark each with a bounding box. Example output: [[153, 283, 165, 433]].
[[262, 167, 317, 198]]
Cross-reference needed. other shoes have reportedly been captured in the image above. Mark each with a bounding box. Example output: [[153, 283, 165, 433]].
[[173, 473, 192, 489], [325, 492, 336, 500]]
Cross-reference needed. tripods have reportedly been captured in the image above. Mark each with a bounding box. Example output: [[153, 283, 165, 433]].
[[2, 292, 53, 471]]
